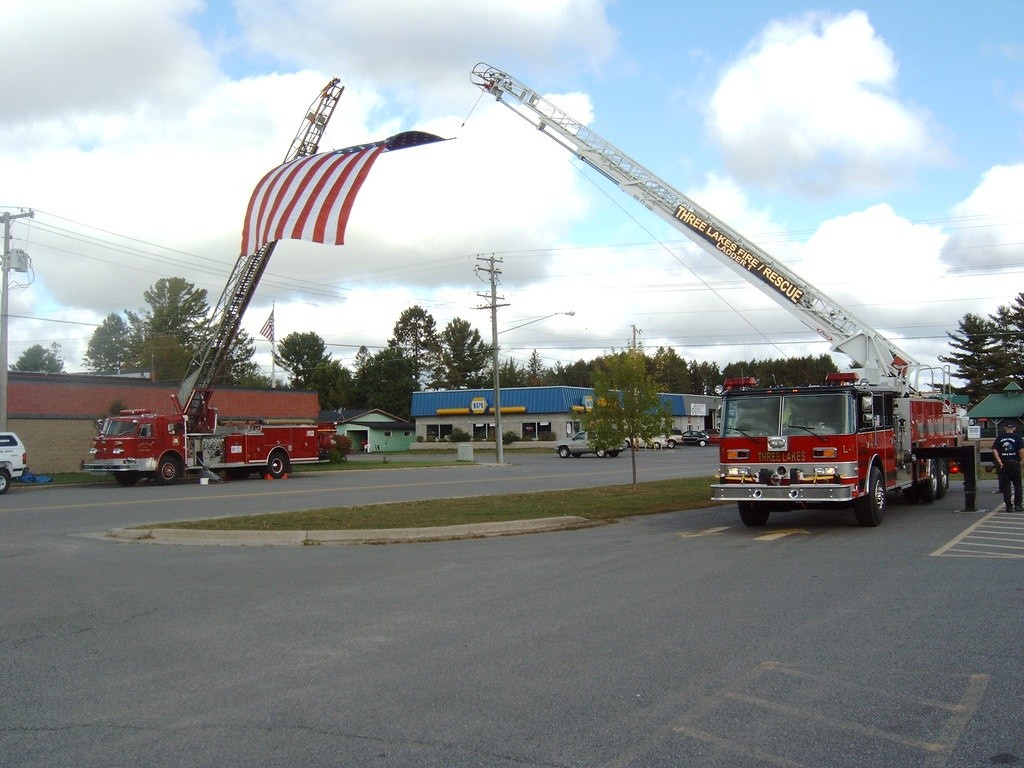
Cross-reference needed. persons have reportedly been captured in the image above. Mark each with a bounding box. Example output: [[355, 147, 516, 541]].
[[991, 421, 1023, 511]]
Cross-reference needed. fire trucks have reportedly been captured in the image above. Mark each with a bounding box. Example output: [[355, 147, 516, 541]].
[[468, 62, 960, 528], [78, 75, 347, 490]]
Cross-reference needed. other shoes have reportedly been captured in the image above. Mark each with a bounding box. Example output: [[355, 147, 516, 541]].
[[1015, 505, 1024, 511], [1006, 504, 1013, 512]]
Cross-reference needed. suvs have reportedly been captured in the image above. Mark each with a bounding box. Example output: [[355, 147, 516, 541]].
[[0, 431, 26, 494]]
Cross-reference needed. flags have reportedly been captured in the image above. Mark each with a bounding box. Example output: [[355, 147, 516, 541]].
[[239, 130, 454, 258], [260, 310, 274, 344]]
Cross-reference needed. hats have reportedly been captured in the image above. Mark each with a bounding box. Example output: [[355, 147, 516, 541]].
[[1005, 420, 1016, 428]]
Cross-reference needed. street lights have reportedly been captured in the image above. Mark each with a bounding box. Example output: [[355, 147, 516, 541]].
[[491, 312, 576, 464]]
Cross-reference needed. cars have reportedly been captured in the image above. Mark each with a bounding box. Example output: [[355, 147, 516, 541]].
[[554, 428, 627, 458], [682, 430, 710, 447], [701, 428, 721, 444], [624, 426, 685, 449]]
